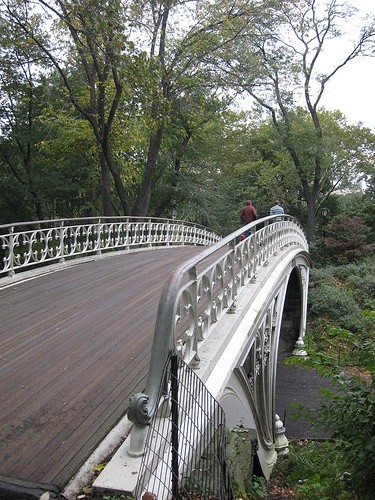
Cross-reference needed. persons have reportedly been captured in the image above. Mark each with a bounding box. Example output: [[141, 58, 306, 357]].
[[241, 200, 257, 237], [270, 200, 284, 223]]
